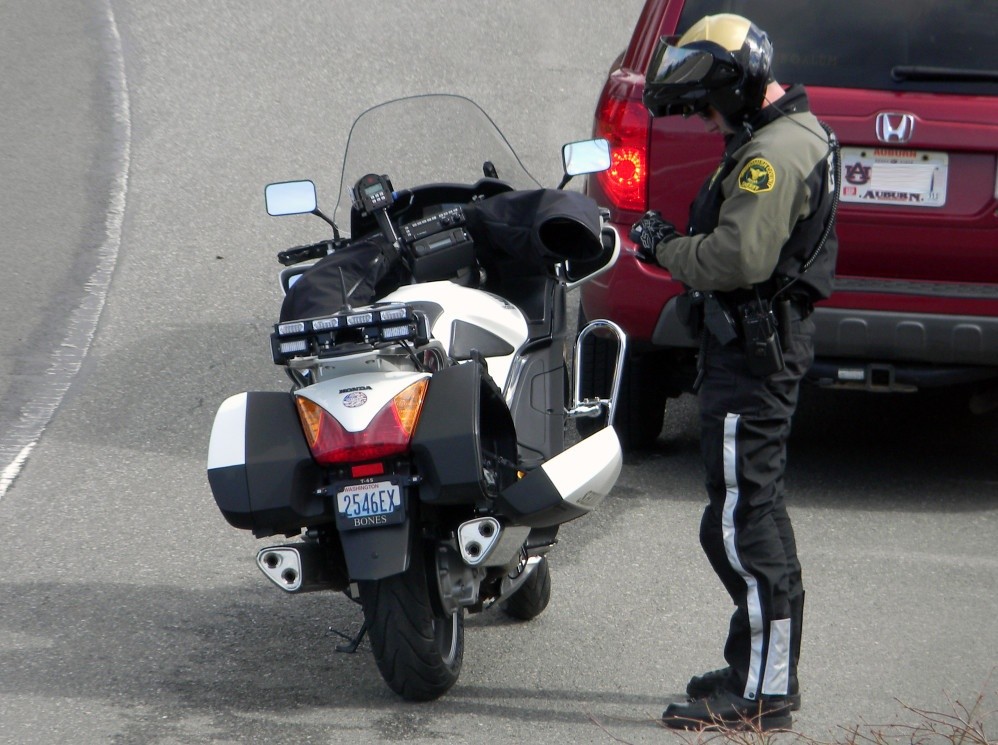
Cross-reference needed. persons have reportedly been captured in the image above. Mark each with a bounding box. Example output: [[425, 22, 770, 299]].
[[628, 13, 840, 730]]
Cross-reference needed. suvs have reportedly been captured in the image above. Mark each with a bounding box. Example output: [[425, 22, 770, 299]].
[[576, 2, 998, 460]]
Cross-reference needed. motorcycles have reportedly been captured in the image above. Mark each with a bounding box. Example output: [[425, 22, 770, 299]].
[[206, 93, 628, 703]]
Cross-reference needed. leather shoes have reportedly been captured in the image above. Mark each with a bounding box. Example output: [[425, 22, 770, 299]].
[[662, 689, 793, 732], [686, 666, 802, 711]]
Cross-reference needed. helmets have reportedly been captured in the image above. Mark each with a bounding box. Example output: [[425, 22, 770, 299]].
[[641, 13, 773, 130]]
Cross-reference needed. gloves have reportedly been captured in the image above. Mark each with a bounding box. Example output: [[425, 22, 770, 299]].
[[630, 210, 676, 266]]
